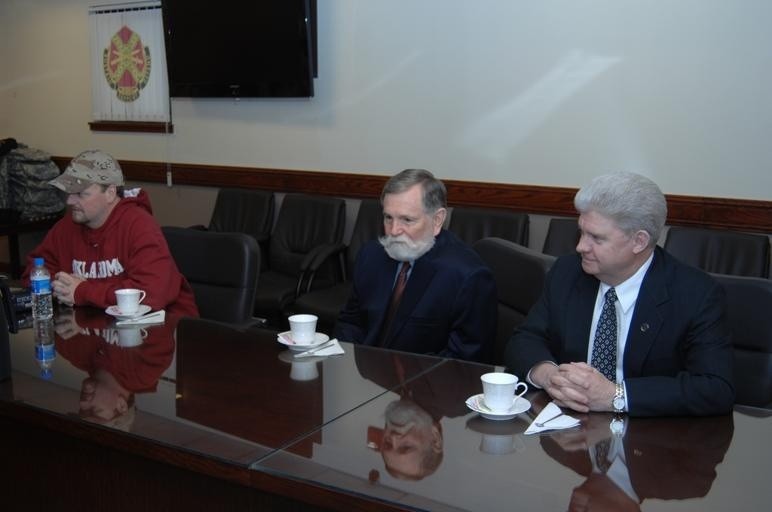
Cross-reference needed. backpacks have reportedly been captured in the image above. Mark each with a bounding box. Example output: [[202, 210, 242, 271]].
[[0, 147, 66, 217]]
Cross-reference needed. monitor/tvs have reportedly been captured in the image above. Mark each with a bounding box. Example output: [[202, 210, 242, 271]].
[[161, 0, 318, 99]]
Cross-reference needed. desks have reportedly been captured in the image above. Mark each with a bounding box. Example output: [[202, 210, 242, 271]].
[[2, 301, 771, 512]]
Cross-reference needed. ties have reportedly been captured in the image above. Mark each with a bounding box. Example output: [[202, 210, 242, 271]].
[[382, 261, 411, 331], [591, 287, 618, 384], [596, 438, 610, 471]]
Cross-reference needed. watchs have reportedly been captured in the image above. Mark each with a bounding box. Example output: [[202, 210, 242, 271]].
[[612, 382, 625, 414], [609, 417, 626, 440]]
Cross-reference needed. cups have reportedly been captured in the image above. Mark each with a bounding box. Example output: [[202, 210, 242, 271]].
[[480, 435, 514, 454], [480, 372, 528, 411], [114, 288, 146, 314], [288, 314, 318, 344], [116, 328, 148, 348], [287, 361, 319, 381]]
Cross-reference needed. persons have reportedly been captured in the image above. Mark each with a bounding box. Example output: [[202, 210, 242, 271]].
[[526, 390, 735, 512], [498, 172, 735, 416], [349, 343, 483, 483], [55, 306, 178, 432], [20, 149, 199, 314], [330, 169, 502, 364]]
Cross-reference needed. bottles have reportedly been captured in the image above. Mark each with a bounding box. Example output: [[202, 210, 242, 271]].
[[32, 319, 58, 380], [29, 258, 53, 319]]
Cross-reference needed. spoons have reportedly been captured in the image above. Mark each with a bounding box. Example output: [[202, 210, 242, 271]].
[[534, 413, 564, 427], [307, 342, 335, 355]]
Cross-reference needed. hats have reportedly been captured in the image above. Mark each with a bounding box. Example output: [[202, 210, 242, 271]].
[[48, 151, 125, 194], [86, 404, 136, 429]]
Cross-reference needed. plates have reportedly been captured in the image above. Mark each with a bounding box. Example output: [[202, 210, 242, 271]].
[[104, 305, 152, 321], [467, 415, 530, 435], [279, 348, 327, 364], [277, 332, 330, 352], [465, 392, 532, 419]]
